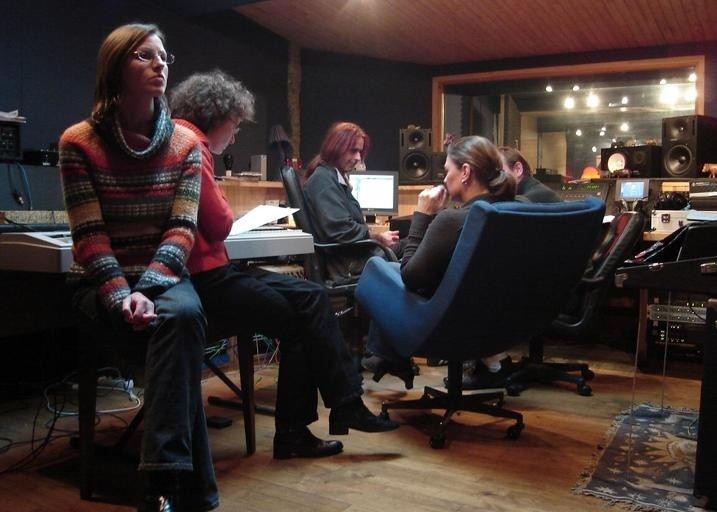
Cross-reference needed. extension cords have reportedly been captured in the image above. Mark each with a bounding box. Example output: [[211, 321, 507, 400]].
[[97, 376, 134, 389]]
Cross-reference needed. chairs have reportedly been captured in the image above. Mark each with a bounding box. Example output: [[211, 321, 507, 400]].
[[354, 195, 606, 449], [505, 210, 646, 398], [282, 166, 399, 373]]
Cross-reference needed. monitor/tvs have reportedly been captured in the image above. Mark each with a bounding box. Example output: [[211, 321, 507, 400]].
[[348, 171, 400, 217]]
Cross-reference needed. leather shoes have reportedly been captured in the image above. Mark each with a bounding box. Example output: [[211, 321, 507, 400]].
[[273, 354, 512, 459]]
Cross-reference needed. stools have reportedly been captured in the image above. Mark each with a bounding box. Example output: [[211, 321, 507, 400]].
[[76, 313, 254, 500]]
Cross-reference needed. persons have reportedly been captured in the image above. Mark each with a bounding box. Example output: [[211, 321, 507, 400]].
[[442, 146, 565, 390], [302, 122, 450, 377], [168, 69, 401, 461], [360, 135, 531, 389], [59, 22, 218, 512]]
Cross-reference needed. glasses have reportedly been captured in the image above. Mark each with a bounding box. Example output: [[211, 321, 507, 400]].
[[131, 49, 175, 64]]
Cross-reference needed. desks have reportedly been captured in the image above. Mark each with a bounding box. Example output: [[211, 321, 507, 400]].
[[0, 222, 315, 273], [218, 177, 440, 223]]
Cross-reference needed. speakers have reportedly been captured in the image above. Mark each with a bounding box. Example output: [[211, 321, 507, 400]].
[[432, 151, 447, 183], [661, 115, 716, 178], [398, 127, 434, 153], [398, 149, 437, 185], [600, 147, 662, 179]]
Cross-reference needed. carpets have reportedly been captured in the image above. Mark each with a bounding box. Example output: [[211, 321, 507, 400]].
[[570, 400, 710, 512]]
